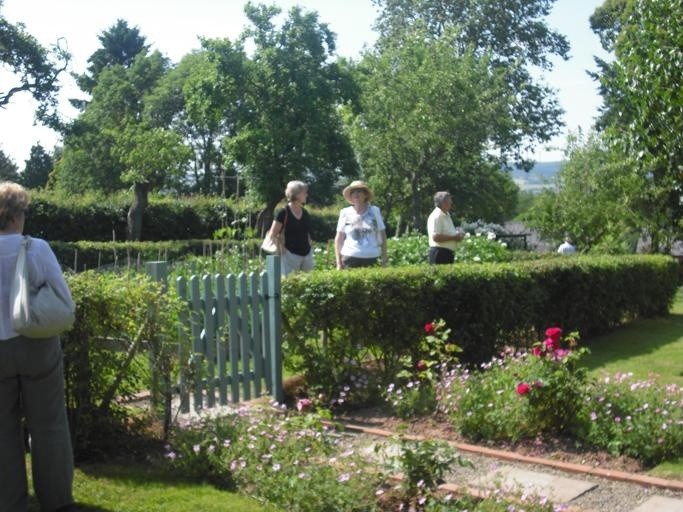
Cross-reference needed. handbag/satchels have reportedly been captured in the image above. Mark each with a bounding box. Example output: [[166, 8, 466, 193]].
[[260, 206, 287, 254], [8, 233, 75, 339]]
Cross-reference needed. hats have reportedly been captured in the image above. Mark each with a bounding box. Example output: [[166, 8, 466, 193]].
[[342, 180, 373, 204]]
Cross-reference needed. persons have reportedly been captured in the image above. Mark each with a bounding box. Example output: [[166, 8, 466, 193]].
[[426, 190, 466, 264], [557, 237, 575, 254], [0, 181, 78, 511], [333, 179, 388, 272], [266, 179, 315, 278]]
